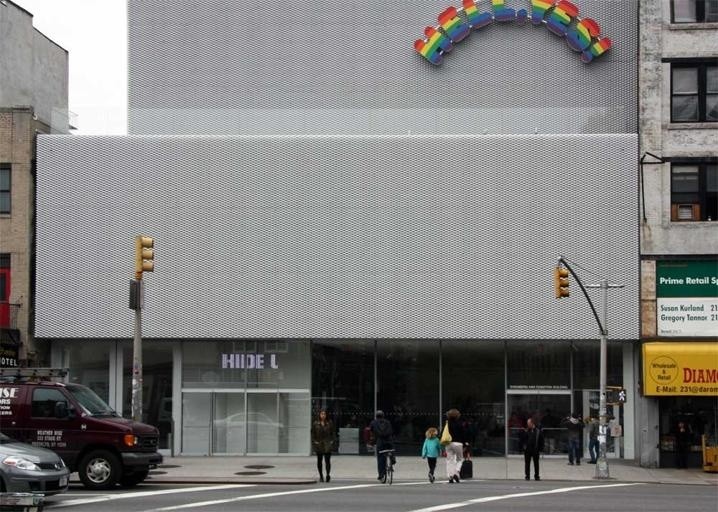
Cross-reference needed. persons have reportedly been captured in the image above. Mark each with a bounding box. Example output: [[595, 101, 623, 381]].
[[472, 405, 585, 481], [674, 419, 690, 468], [367, 408, 397, 481], [311, 409, 340, 483], [444, 409, 472, 482], [346, 414, 359, 427], [587, 414, 600, 464], [421, 427, 443, 485]]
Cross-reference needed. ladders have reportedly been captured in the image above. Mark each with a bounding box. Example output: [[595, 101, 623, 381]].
[[0, 367, 70, 377]]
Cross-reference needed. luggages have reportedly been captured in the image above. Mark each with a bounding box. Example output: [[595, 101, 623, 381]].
[[461, 446, 472, 479]]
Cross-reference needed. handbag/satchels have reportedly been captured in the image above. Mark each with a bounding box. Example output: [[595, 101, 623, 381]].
[[440, 424, 452, 444]]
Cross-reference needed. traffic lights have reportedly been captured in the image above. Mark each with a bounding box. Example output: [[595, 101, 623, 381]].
[[553, 266, 570, 301], [611, 388, 627, 403], [134, 235, 155, 279]]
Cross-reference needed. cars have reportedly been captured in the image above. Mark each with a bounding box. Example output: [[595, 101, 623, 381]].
[[0, 430, 73, 503], [213, 409, 285, 435]]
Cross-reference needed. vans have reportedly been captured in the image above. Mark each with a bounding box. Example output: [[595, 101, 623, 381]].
[[0, 364, 166, 492]]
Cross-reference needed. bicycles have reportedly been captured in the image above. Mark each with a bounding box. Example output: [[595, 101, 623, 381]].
[[377, 448, 394, 485]]
